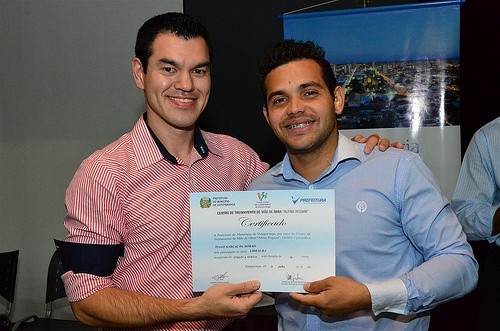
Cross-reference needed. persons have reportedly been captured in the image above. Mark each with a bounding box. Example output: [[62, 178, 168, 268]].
[[450, 116, 500, 331], [247, 39, 480, 331], [54, 12, 405, 331]]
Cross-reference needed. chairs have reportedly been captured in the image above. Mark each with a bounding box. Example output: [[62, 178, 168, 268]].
[[0, 250, 20, 331], [12, 246, 98, 331]]
